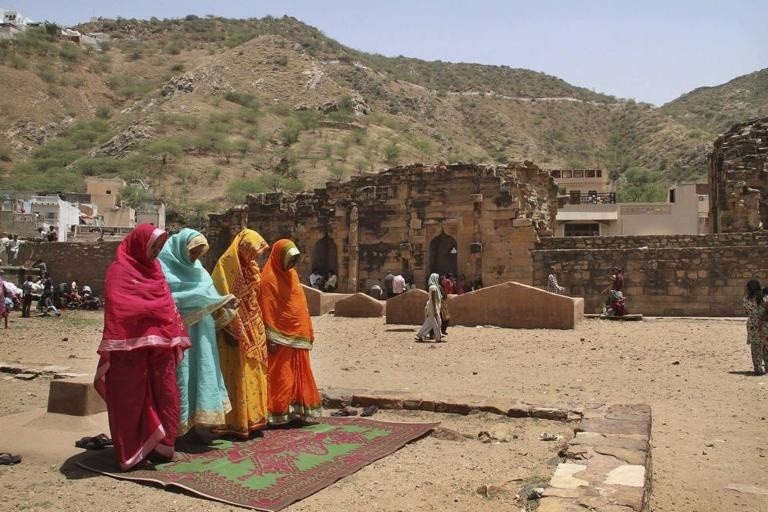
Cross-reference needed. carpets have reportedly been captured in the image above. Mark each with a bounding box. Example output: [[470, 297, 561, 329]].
[[75, 416, 442, 512]]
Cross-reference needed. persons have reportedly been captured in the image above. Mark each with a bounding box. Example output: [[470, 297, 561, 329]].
[[740, 279, 768, 376], [208, 229, 271, 440], [159, 226, 244, 449], [547, 264, 566, 296], [0, 225, 105, 328], [599, 273, 617, 319], [93, 226, 195, 469], [611, 265, 628, 314], [309, 268, 476, 343], [259, 240, 325, 428]]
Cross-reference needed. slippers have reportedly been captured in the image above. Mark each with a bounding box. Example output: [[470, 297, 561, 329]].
[[74, 429, 113, 449], [0, 449, 23, 465]]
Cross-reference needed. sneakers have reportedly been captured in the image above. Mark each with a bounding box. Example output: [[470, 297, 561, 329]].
[[414, 334, 445, 346]]
